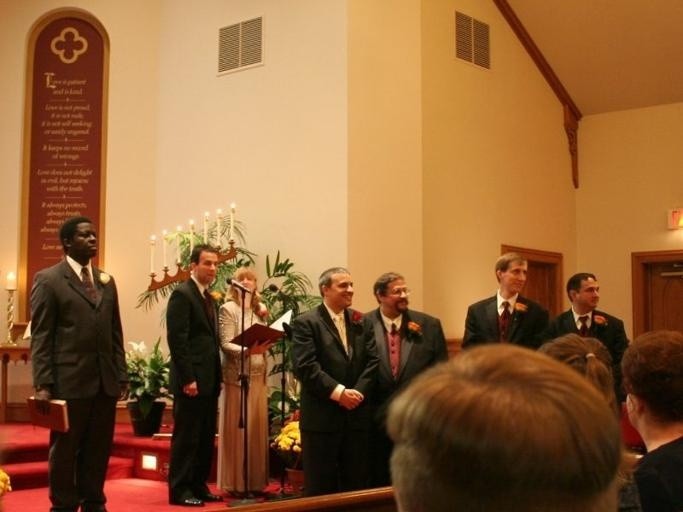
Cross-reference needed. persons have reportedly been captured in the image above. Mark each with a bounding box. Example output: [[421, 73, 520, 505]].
[[385, 343, 621, 512], [548, 272, 629, 406], [29, 215, 132, 512], [460, 251, 548, 352], [165, 243, 222, 507], [533, 333, 641, 471], [289, 267, 381, 495], [215, 266, 271, 500], [620, 329, 683, 512], [362, 271, 449, 488]]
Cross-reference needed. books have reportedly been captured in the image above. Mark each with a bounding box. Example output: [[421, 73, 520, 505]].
[[229, 309, 293, 349], [26, 396, 70, 433]]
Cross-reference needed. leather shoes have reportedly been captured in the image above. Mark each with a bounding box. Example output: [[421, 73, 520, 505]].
[[183, 494, 222, 505]]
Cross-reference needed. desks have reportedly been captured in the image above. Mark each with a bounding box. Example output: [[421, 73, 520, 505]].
[[0, 343, 34, 425]]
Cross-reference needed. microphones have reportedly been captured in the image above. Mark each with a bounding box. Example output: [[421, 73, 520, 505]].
[[269, 285, 287, 301], [225, 277, 251, 293]]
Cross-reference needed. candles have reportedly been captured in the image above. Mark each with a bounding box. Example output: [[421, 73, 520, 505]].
[[148, 199, 235, 273], [6, 270, 17, 289]]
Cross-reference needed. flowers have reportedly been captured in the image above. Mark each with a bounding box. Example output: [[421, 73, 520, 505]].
[[121, 335, 170, 421], [268, 420, 307, 470]]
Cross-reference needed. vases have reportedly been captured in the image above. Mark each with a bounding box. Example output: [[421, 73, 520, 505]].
[[284, 466, 305, 493], [126, 400, 166, 436]]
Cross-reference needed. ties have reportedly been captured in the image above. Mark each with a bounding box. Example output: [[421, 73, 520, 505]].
[[203, 291, 215, 320], [82, 268, 97, 301], [501, 302, 510, 339], [579, 316, 588, 332]]
